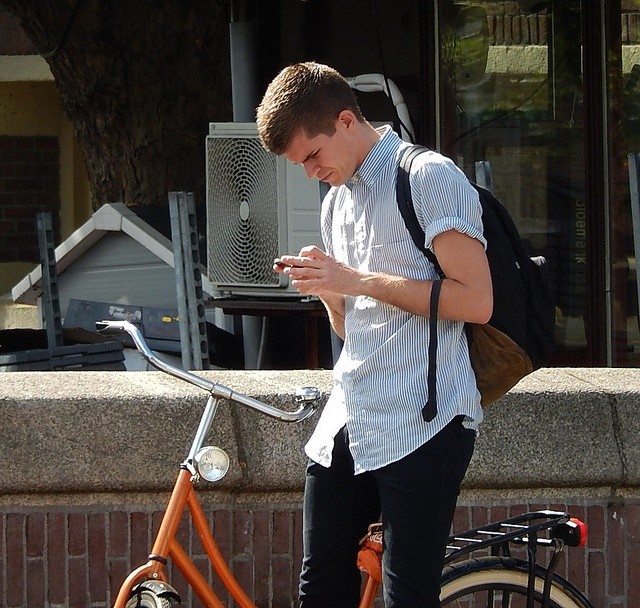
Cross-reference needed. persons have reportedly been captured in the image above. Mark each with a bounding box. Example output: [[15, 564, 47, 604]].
[[255, 61, 494, 607]]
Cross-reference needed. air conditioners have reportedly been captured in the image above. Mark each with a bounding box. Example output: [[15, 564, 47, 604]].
[[203, 120, 397, 300]]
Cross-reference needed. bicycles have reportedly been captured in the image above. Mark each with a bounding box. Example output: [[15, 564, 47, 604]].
[[95, 320, 588, 602]]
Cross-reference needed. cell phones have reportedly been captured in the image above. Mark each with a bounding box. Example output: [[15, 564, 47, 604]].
[[274, 258, 304, 269]]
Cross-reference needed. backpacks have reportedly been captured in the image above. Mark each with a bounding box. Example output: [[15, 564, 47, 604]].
[[394, 141, 548, 424]]
